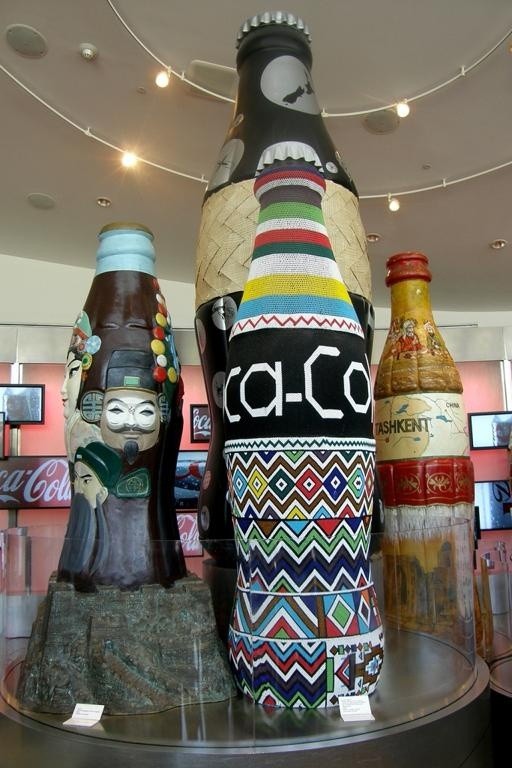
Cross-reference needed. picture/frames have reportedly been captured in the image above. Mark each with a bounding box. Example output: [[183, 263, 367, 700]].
[[190, 404, 210, 442], [0, 454, 76, 509], [467, 411, 512, 450], [174, 449, 208, 509], [176, 510, 203, 558], [474, 479, 512, 531]]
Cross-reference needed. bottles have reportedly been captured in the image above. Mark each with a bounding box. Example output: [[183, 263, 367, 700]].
[[226, 142, 380, 711], [193, 10, 384, 552], [374, 254, 479, 669], [62, 220, 184, 584]]
